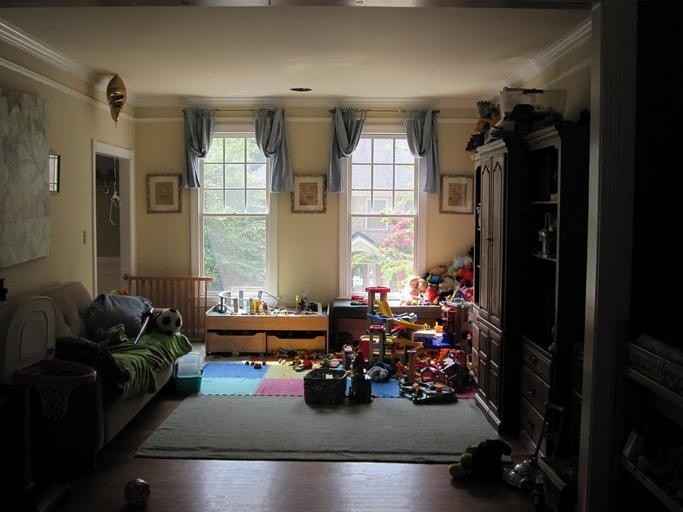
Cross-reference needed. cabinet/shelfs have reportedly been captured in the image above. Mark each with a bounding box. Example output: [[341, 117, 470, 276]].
[[618, 328, 682, 512], [204, 302, 330, 357]]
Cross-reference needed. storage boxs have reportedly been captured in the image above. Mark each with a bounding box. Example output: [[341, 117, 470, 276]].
[[172, 364, 206, 394], [499, 85, 567, 118]]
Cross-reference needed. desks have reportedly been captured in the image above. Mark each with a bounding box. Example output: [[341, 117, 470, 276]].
[[330, 298, 444, 349]]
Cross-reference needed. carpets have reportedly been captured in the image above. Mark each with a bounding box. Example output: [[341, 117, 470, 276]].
[[134, 394, 514, 465]]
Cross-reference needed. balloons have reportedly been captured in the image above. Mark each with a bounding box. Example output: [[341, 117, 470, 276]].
[[105, 74, 127, 130]]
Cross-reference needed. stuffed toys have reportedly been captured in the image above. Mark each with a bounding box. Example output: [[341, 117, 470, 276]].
[[447, 437, 512, 492]]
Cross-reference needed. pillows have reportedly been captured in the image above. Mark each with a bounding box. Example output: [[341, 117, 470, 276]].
[[57, 335, 121, 408]]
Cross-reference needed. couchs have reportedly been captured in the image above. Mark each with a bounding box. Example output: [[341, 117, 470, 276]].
[[0, 279, 192, 445]]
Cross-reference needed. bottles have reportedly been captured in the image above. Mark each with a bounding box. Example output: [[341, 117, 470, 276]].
[[293, 292, 312, 316], [247, 298, 287, 316]]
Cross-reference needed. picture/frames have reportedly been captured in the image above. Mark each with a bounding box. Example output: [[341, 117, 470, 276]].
[[289, 173, 327, 215], [146, 172, 182, 214], [439, 173, 474, 215]]
